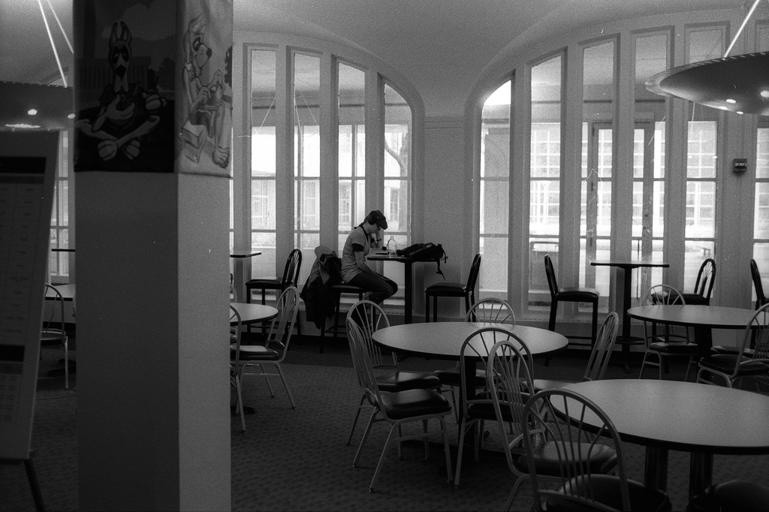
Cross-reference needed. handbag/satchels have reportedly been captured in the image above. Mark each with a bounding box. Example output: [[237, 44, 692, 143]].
[[396, 242, 443, 260]]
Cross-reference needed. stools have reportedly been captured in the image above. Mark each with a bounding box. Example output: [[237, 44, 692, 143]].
[[317, 247, 367, 354], [542, 252, 600, 366], [650, 258, 719, 344], [245, 248, 305, 341], [423, 252, 482, 323], [748, 258, 769, 348]]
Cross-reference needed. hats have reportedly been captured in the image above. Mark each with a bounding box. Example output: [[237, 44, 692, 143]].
[[372, 210, 387, 229]]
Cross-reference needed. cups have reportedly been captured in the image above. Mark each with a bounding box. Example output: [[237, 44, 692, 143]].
[[388, 243, 399, 258]]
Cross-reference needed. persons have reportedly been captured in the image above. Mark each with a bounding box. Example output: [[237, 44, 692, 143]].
[[342, 210, 398, 304]]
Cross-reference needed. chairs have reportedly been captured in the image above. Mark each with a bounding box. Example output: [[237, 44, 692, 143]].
[[486, 340, 623, 512], [41, 284, 71, 390], [229, 286, 302, 414], [521, 385, 673, 512], [639, 283, 699, 382], [454, 327, 547, 487], [522, 311, 619, 401], [346, 300, 443, 457], [229, 302, 249, 432], [685, 477, 768, 510], [435, 296, 518, 423], [345, 317, 453, 494], [697, 302, 768, 389]]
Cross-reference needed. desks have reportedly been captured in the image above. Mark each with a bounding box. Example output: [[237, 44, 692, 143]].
[[546, 374, 768, 491], [229, 248, 264, 263], [367, 248, 447, 326], [45, 282, 74, 304], [588, 256, 672, 364], [229, 301, 279, 327]]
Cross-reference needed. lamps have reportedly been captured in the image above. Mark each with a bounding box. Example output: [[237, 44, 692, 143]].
[[642, 0, 769, 119], [1, 0, 78, 136]]
